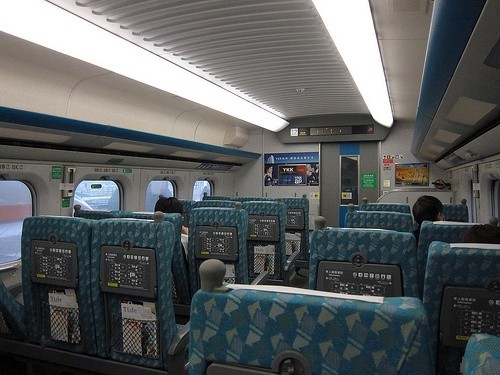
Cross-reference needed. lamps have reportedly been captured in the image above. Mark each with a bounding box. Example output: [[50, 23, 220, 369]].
[[311, 0, 394, 127], [0, 0, 290, 132]]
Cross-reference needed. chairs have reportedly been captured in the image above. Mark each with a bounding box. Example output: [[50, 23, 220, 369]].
[[1, 196, 500, 375]]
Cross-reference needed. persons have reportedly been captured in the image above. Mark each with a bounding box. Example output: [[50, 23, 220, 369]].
[[412, 195, 445, 245], [264, 165, 274, 186], [464, 223, 500, 245], [154, 196, 188, 257]]
